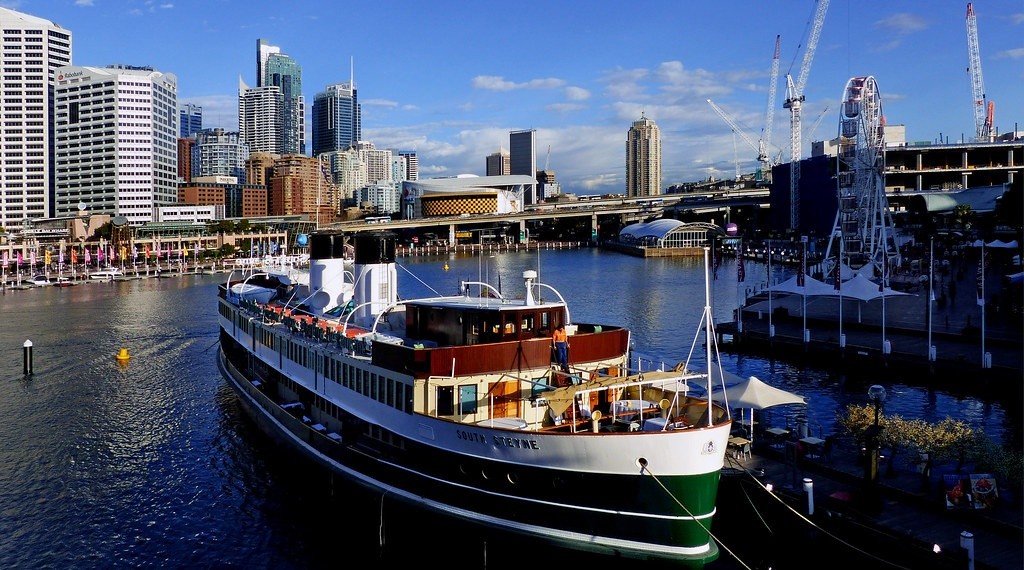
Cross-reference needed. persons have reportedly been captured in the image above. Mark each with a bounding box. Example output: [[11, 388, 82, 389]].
[[553, 324, 570, 374]]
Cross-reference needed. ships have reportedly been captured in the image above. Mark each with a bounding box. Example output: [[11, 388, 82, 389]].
[[216, 228, 732, 570]]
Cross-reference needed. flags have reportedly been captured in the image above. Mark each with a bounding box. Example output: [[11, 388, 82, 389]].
[[110, 246, 116, 260], [2, 251, 9, 268], [17, 251, 22, 266], [45, 250, 50, 264], [195, 243, 198, 254], [797, 241, 805, 287], [85, 248, 90, 262], [58, 249, 64, 262], [145, 246, 150, 258], [168, 244, 172, 257], [711, 242, 719, 279], [132, 245, 138, 258], [877, 236, 890, 291], [975, 242, 989, 306], [833, 237, 843, 291], [71, 248, 77, 263], [766, 243, 771, 288], [737, 244, 746, 282], [157, 245, 162, 257], [184, 245, 188, 256], [97, 247, 104, 261], [122, 246, 127, 260], [929, 242, 936, 300], [30, 251, 36, 265]]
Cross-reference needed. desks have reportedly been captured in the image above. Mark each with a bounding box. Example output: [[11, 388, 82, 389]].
[[610, 399, 660, 421], [643, 417, 681, 431], [476, 417, 528, 430]]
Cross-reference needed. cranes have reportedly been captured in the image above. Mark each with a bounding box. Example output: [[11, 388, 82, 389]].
[[963, 2, 998, 141], [782, 1, 830, 238], [705, 33, 779, 184]]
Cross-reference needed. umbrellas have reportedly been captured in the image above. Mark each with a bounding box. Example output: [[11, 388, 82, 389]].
[[698, 376, 808, 448], [630, 351, 746, 390]]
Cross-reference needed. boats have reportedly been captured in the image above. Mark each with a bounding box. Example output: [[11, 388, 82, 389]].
[[0, 271, 143, 289]]
